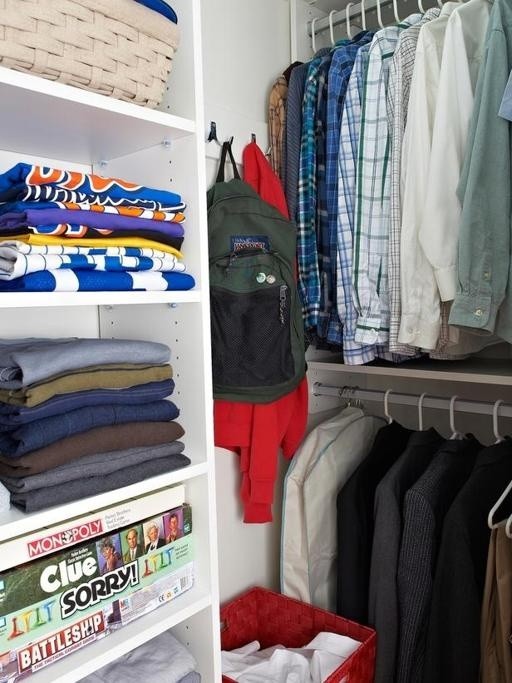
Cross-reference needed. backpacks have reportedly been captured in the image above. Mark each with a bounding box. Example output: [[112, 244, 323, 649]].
[[206, 140, 308, 403]]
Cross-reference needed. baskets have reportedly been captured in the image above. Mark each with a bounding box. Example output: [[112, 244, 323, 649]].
[[0, 0, 181, 110]]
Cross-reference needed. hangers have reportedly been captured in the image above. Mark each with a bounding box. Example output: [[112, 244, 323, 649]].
[[338, 383, 512, 539], [306, 0, 445, 53]]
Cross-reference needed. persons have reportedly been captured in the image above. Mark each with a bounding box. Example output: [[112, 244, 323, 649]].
[[123, 529, 143, 564], [166, 512, 183, 543], [99, 537, 122, 574], [145, 521, 164, 553]]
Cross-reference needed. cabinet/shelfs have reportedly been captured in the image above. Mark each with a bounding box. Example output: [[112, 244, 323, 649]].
[[0, 0, 221, 683], [290, 1, 512, 419]]
